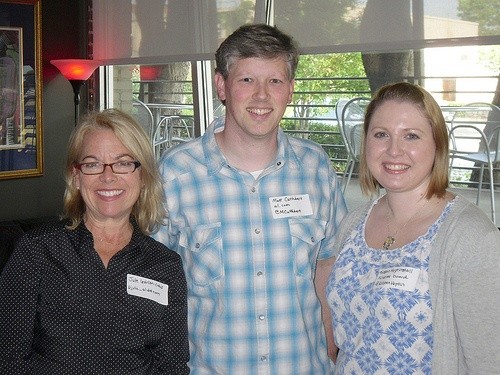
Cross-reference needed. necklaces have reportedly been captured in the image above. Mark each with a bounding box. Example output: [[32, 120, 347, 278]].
[[382, 198, 429, 250], [92, 232, 128, 246]]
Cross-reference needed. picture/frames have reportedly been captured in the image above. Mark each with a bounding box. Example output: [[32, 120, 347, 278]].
[[0, 0, 46, 180]]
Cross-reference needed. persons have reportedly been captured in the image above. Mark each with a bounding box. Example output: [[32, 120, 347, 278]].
[[0, 108, 191, 375], [146, 23, 349, 375], [324, 82, 500, 375]]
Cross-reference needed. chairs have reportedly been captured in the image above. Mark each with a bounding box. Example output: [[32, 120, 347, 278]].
[[133, 97, 172, 160], [451, 102, 500, 206], [169, 97, 226, 148], [335, 99, 366, 191], [341, 96, 375, 198], [448, 125, 500, 224]]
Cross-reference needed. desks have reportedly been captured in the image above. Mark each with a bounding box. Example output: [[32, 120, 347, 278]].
[[442, 106, 493, 176], [133, 103, 194, 161]]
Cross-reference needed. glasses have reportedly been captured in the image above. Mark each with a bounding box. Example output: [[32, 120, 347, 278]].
[[74, 160, 141, 176]]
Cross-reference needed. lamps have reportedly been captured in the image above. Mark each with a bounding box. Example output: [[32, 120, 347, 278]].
[[49, 59, 105, 128]]
